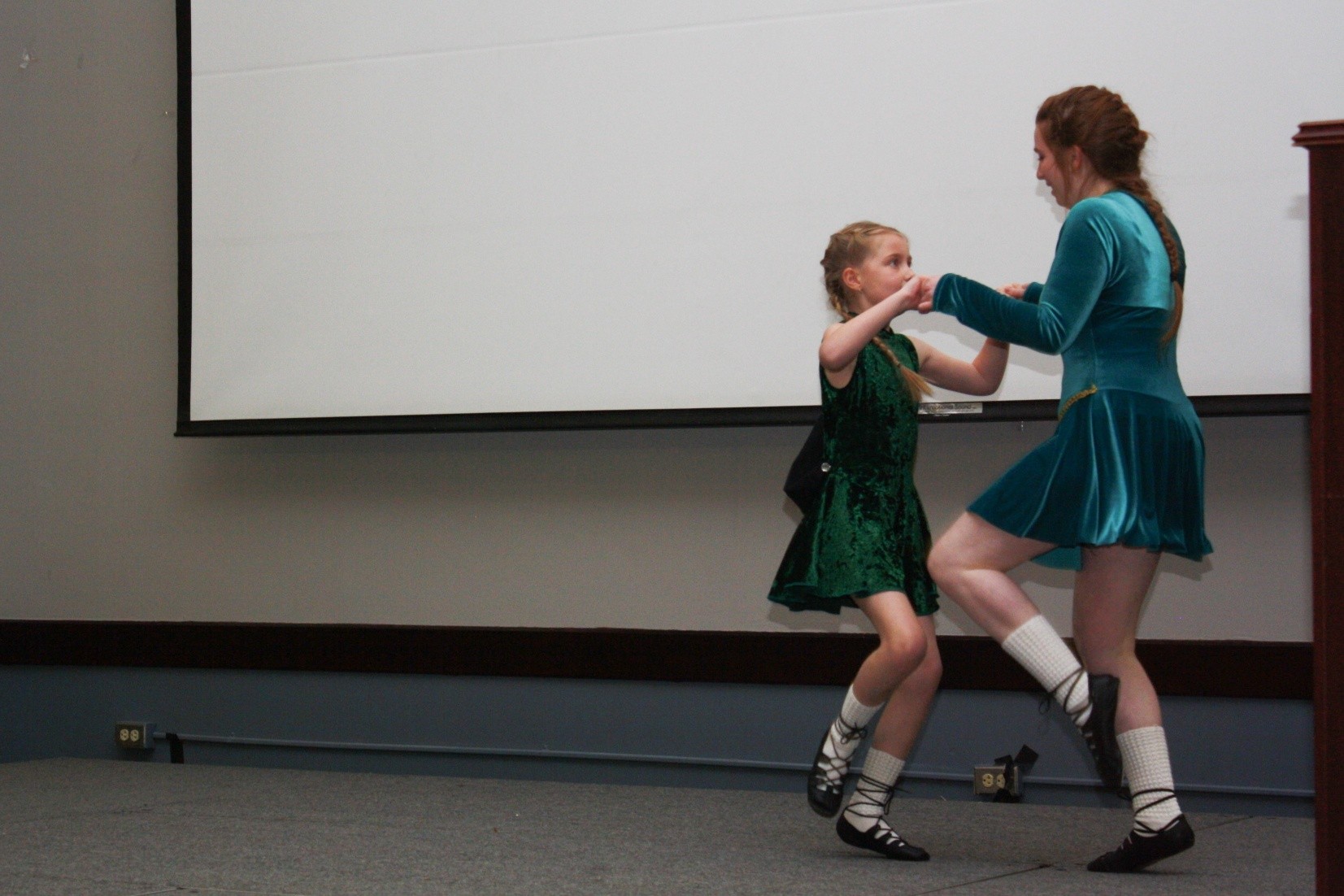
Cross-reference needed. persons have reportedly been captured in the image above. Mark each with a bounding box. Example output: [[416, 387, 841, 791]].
[[764, 221, 1021, 859], [915, 84, 1214, 872]]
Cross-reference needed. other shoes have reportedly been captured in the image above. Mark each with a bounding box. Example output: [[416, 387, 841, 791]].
[[807, 716, 868, 818], [1048, 664, 1125, 795], [1087, 787, 1196, 874], [836, 772, 932, 861]]
